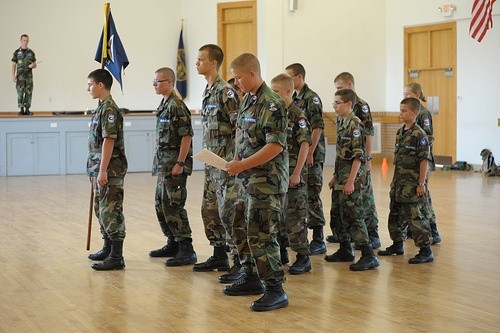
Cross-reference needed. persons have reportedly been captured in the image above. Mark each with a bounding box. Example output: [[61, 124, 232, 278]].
[[228, 78, 246, 99], [86, 69, 128, 270], [11, 34, 37, 115], [193, 44, 240, 284], [325, 72, 381, 251], [401, 82, 442, 246], [376, 98, 436, 264], [271, 75, 312, 275], [324, 89, 381, 271], [148, 67, 198, 266], [284, 64, 328, 255], [222, 53, 290, 312]]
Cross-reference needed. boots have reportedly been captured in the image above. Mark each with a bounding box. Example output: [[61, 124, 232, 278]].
[[326, 233, 351, 243], [409, 247, 434, 264], [288, 252, 311, 275], [349, 245, 380, 270], [377, 241, 404, 256], [430, 227, 441, 243], [223, 276, 265, 296], [17, 107, 33, 115], [249, 283, 288, 312], [193, 246, 231, 272], [355, 232, 381, 250], [88, 238, 126, 271], [308, 227, 327, 255], [280, 248, 289, 265], [218, 255, 251, 283], [164, 237, 197, 266], [323, 245, 355, 262], [149, 237, 180, 257]]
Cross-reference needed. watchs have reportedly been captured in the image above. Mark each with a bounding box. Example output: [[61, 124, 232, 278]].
[[418, 183, 424, 186], [176, 161, 185, 166]]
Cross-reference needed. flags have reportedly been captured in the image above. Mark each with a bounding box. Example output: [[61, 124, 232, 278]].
[[94, 10, 130, 94], [468, 0, 496, 43], [176, 30, 188, 100]]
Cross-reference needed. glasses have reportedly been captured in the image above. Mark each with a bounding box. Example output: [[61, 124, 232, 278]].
[[332, 99, 352, 105], [152, 79, 170, 83]]
